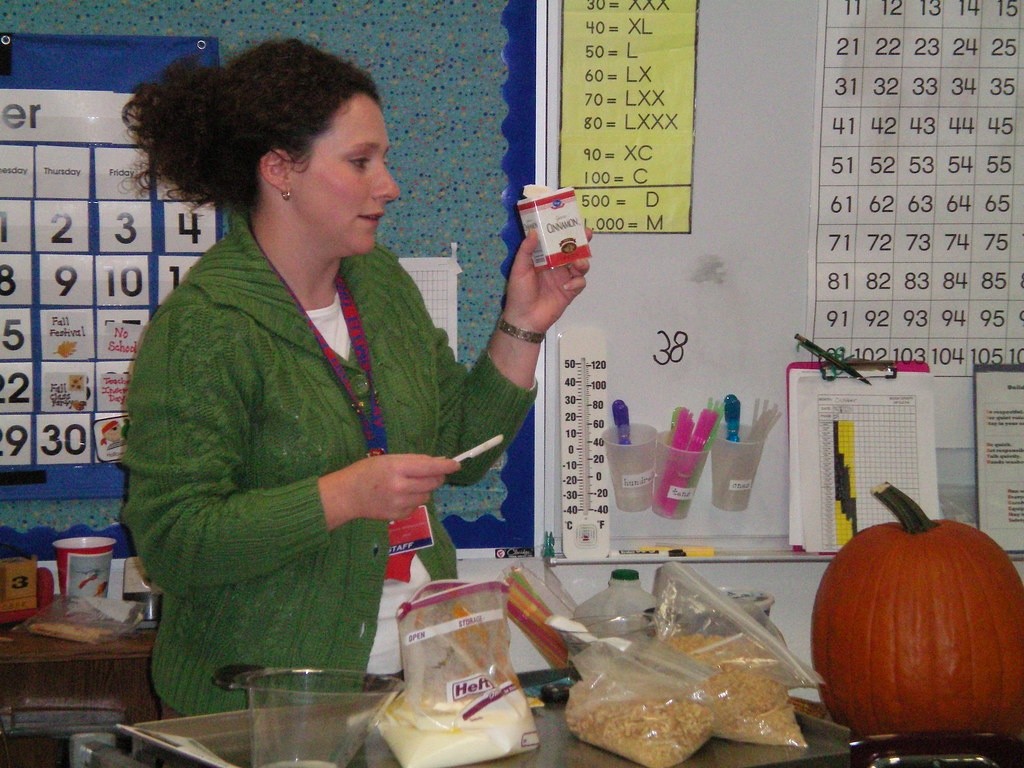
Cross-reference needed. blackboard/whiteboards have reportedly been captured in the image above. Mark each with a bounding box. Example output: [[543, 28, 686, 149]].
[[534, 1, 1024, 567]]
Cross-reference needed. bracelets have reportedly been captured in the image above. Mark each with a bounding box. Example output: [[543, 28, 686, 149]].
[[496, 313, 547, 345]]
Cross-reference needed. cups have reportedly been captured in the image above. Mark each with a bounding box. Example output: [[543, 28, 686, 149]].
[[210, 665, 406, 768], [710, 425, 765, 511], [52, 536, 116, 596], [676, 588, 774, 632], [603, 422, 657, 513], [655, 430, 711, 520], [556, 614, 658, 657]]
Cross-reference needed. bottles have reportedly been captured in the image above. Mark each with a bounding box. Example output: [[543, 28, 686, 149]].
[[571, 570, 660, 617]]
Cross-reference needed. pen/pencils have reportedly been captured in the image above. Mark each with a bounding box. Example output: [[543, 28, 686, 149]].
[[451, 434, 504, 463], [794, 333, 872, 386]]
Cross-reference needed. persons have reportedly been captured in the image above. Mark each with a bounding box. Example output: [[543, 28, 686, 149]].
[[115, 39, 594, 727]]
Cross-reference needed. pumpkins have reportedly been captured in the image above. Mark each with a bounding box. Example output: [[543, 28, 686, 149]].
[[811, 481, 1024, 741]]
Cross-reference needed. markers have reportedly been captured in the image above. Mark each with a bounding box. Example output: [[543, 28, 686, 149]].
[[619, 549, 686, 557]]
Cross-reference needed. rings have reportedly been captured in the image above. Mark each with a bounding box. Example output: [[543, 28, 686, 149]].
[[567, 263, 573, 269]]
